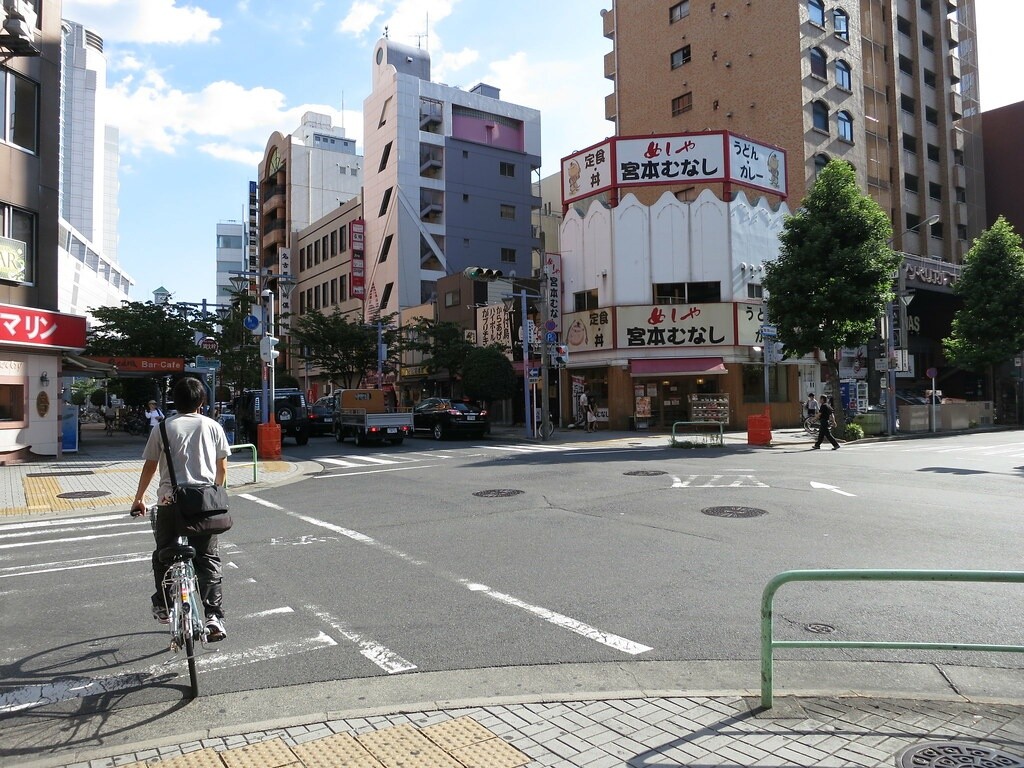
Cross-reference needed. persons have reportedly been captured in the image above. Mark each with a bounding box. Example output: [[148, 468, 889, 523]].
[[587, 396, 596, 433], [574, 387, 590, 431], [811, 394, 840, 450], [145, 400, 165, 439], [803, 393, 819, 431], [130, 377, 232, 644], [104, 401, 116, 431]]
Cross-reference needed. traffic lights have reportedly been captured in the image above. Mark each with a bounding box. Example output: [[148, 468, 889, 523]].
[[878, 343, 886, 358], [555, 345, 569, 367], [464, 267, 503, 282], [260, 336, 279, 362]]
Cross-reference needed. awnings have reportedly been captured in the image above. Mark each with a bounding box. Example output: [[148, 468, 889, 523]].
[[630, 359, 728, 378]]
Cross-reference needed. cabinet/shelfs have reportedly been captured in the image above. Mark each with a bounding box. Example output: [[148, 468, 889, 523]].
[[688, 392, 730, 423]]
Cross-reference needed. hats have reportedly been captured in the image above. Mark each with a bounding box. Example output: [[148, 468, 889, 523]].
[[148, 400, 157, 404]]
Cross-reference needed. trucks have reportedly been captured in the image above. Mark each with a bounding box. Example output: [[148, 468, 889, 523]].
[[332, 389, 414, 446]]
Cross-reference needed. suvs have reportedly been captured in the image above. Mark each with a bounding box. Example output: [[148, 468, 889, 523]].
[[234, 388, 308, 445], [409, 397, 489, 441]]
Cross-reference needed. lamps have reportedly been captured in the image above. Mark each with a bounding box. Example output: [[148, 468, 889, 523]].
[[475, 303, 487, 307], [662, 376, 669, 386], [39, 371, 49, 388], [484, 300, 497, 306], [467, 305, 481, 310], [492, 295, 505, 303], [697, 375, 704, 384]]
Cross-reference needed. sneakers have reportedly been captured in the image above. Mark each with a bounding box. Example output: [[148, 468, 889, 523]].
[[205, 613, 227, 641], [151, 605, 173, 624]]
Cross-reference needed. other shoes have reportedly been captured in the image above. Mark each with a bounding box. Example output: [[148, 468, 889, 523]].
[[812, 446, 820, 449], [832, 445, 840, 450]]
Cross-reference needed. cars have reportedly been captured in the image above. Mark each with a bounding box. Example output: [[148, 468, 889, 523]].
[[166, 402, 177, 415], [308, 405, 335, 433], [313, 396, 337, 405]]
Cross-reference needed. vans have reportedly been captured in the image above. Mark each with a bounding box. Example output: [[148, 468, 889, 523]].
[[215, 402, 227, 407]]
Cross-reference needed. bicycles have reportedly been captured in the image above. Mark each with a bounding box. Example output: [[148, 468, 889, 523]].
[[80, 412, 99, 423], [803, 414, 832, 434], [538, 410, 554, 438], [127, 411, 150, 437], [106, 418, 114, 437], [129, 508, 223, 696]]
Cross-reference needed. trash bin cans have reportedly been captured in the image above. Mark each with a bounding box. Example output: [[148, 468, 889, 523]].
[[747, 415, 772, 446], [257, 423, 282, 459], [853, 414, 881, 434]]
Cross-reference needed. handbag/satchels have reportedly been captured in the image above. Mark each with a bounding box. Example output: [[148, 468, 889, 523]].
[[179, 485, 232, 536], [805, 400, 809, 409]]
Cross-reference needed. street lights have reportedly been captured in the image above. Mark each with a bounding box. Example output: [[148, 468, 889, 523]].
[[177, 302, 232, 419], [228, 270, 297, 424], [882, 215, 940, 435]]
